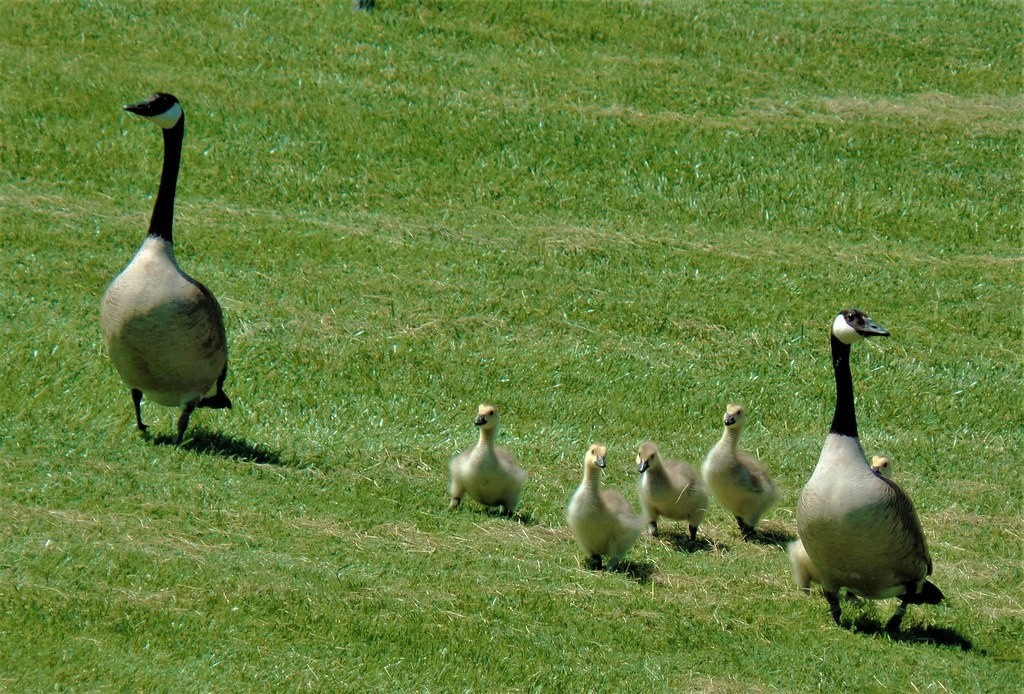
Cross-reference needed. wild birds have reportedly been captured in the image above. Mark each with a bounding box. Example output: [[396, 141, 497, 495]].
[[634, 440, 710, 546], [98, 90, 234, 448], [564, 444, 646, 577], [787, 308, 947, 638], [701, 403, 779, 540], [445, 403, 525, 519]]
